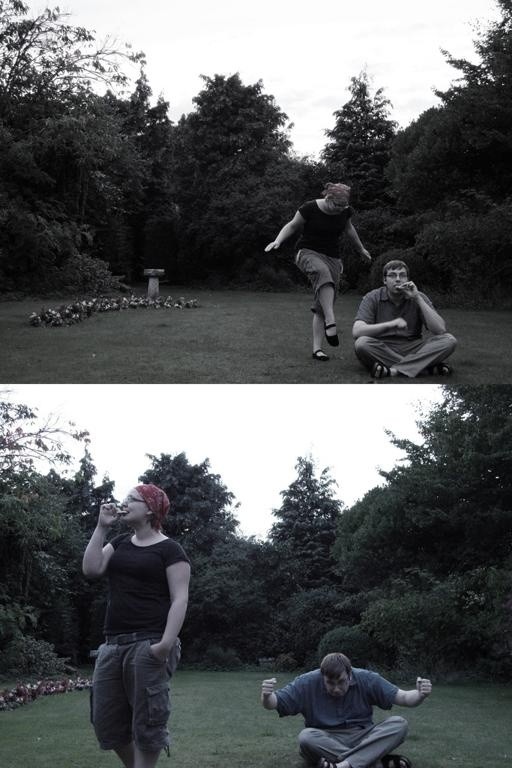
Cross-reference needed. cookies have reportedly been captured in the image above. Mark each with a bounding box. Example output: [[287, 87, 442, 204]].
[[395, 284, 404, 289], [116, 510, 128, 515]]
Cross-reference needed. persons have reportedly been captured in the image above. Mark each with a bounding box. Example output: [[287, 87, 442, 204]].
[[81, 482, 193, 765], [265, 181, 371, 361], [261, 650, 433, 767], [353, 259, 459, 378]]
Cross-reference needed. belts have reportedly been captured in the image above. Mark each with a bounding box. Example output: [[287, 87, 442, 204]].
[[105, 630, 163, 646]]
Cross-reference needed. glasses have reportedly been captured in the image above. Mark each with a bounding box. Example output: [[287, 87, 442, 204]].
[[330, 198, 350, 209], [122, 496, 145, 503]]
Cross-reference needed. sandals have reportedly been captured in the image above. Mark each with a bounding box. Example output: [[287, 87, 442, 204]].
[[319, 757, 338, 768], [427, 362, 453, 378], [324, 320, 340, 347], [380, 754, 411, 768], [312, 350, 329, 361], [370, 362, 391, 380]]
